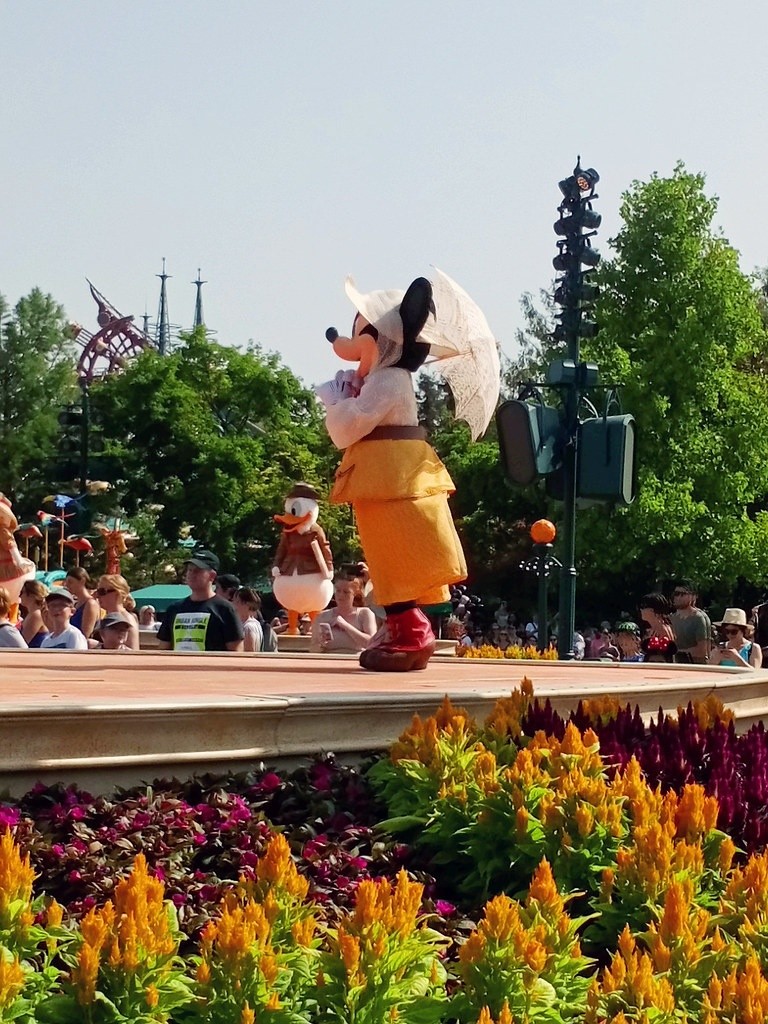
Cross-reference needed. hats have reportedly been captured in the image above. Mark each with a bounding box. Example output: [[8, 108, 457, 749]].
[[102, 612, 134, 627], [676, 578, 698, 594], [712, 608, 755, 636], [183, 550, 220, 573], [641, 637, 678, 662], [216, 574, 240, 590], [45, 588, 74, 604]]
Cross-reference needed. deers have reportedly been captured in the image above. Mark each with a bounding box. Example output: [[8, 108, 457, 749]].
[[97, 526, 134, 576]]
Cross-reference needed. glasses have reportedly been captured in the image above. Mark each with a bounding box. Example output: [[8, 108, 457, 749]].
[[672, 590, 692, 596], [97, 587, 116, 596], [493, 628, 499, 630], [724, 628, 740, 636], [550, 639, 556, 641], [500, 633, 507, 636]]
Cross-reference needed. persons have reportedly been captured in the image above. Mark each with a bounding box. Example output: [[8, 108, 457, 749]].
[[308, 562, 390, 655], [574, 581, 768, 668], [156, 550, 277, 651], [449, 583, 559, 651], [0, 568, 165, 650]]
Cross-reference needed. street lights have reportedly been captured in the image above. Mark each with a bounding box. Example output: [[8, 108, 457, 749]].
[[520, 520, 563, 656]]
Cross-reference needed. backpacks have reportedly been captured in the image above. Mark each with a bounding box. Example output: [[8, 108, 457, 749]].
[[259, 619, 278, 652]]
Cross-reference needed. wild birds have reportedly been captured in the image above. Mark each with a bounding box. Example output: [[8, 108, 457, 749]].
[[36, 510, 77, 527], [57, 534, 103, 557], [41, 492, 89, 511], [17, 520, 43, 539]]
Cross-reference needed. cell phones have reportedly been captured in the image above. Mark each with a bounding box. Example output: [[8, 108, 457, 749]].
[[717, 644, 726, 653], [320, 623, 333, 641]]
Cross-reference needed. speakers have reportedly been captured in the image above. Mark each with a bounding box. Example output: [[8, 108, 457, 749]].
[[579, 412, 638, 505], [495, 400, 561, 486]]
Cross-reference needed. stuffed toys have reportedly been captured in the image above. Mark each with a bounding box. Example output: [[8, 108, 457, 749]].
[[271, 482, 335, 635], [314, 277, 467, 670]]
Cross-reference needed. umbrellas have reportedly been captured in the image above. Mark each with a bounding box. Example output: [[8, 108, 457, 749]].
[[361, 264, 501, 442]]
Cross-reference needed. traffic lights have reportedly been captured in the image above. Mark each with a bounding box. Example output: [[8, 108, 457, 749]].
[[495, 399, 563, 485], [580, 414, 641, 508]]
